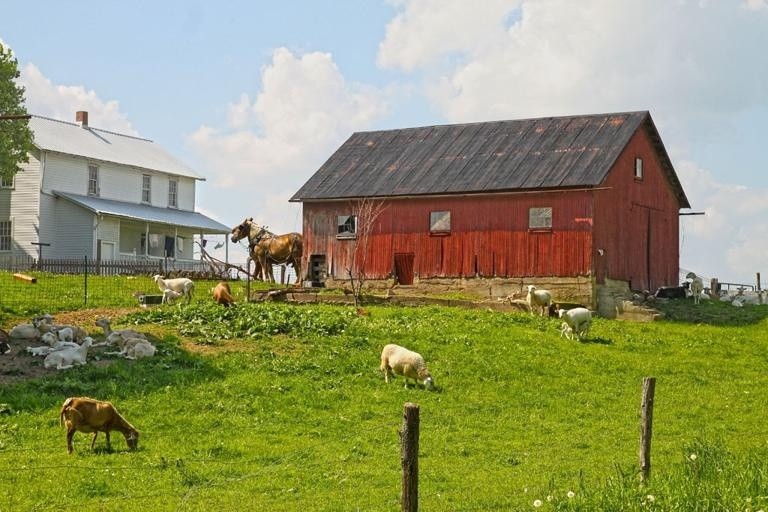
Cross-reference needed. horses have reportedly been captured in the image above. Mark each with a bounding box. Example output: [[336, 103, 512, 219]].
[[231, 218, 304, 286]]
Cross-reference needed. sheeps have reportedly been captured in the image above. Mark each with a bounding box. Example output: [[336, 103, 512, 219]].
[[525, 285, 551, 317], [152, 274, 195, 305], [716, 286, 768, 307], [379, 343, 436, 393], [60, 396, 138, 454], [554, 307, 596, 342], [161, 288, 183, 305], [0, 313, 157, 370], [213, 282, 235, 308], [654, 272, 712, 305]]
